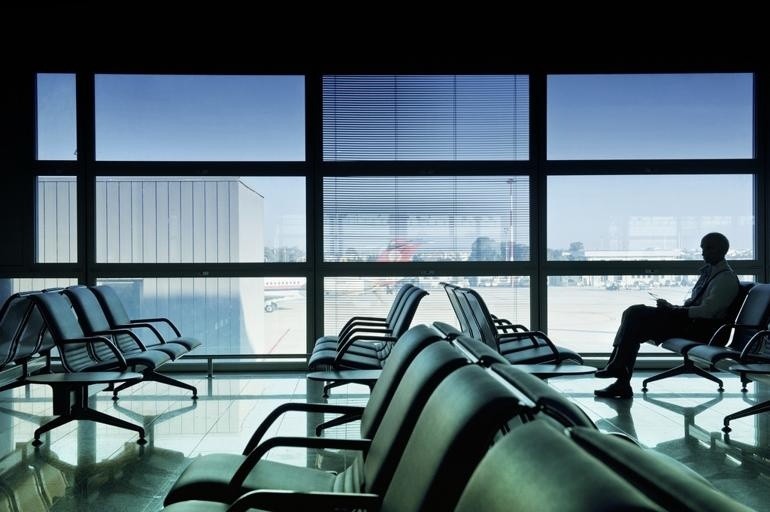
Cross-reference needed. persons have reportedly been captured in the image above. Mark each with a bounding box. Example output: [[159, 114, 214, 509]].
[[592, 232, 740, 397]]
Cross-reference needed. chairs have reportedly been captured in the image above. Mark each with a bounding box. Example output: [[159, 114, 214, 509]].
[[2, 287, 201, 445], [303, 281, 596, 433], [639, 275, 769, 438], [161, 320, 756, 511]]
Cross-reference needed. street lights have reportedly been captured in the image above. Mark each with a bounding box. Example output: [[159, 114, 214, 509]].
[[504, 178, 518, 288]]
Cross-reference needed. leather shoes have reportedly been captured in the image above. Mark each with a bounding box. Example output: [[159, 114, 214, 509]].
[[595, 366, 628, 378], [594, 382, 634, 398]]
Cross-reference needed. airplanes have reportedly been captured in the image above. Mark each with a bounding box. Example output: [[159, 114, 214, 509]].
[[263, 239, 427, 314]]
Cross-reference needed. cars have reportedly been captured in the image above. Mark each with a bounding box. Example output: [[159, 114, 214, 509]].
[[607, 279, 681, 292]]
